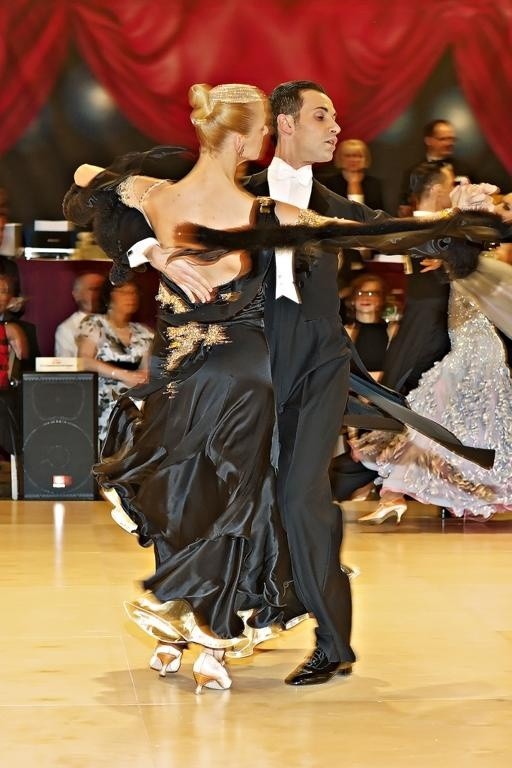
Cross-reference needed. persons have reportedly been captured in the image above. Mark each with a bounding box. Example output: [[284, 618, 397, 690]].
[[0, 265, 29, 396], [317, 118, 512, 524], [54, 271, 155, 439]]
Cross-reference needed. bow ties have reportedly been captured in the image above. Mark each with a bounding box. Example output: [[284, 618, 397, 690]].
[[277, 163, 313, 185]]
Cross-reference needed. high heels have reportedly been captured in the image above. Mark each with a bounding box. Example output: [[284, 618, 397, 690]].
[[191, 651, 233, 695], [356, 496, 408, 526], [147, 640, 184, 678]]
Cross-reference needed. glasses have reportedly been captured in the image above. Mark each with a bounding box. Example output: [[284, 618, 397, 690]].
[[355, 289, 379, 298]]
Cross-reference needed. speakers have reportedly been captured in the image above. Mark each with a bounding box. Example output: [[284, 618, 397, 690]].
[[21, 371, 100, 502]]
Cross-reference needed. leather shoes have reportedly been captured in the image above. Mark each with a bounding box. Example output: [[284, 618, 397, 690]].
[[284, 645, 353, 688]]
